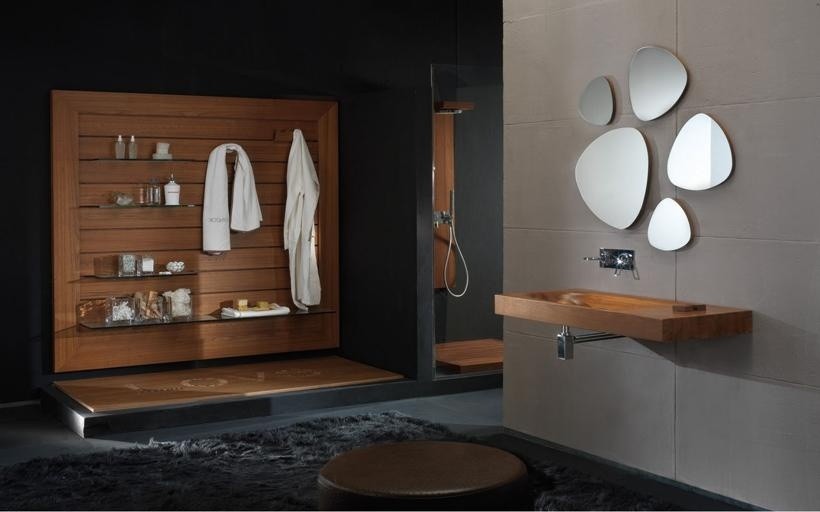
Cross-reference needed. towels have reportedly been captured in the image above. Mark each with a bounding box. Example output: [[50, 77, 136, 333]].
[[221, 307, 291, 319], [201, 143, 262, 258], [282, 128, 325, 313]]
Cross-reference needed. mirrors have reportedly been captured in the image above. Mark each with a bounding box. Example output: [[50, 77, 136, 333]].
[[626, 43, 690, 123], [646, 197, 694, 251], [572, 125, 653, 231], [664, 111, 734, 194], [573, 75, 616, 127]]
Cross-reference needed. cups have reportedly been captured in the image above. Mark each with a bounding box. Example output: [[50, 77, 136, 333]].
[[115, 253, 135, 277], [155, 142, 170, 154], [135, 293, 163, 324], [165, 293, 193, 321], [106, 295, 138, 326], [136, 255, 154, 277]]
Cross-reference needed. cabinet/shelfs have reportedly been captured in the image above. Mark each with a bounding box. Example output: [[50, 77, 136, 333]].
[[49, 88, 338, 374]]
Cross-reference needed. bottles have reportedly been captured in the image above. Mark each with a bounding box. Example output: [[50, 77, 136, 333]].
[[135, 183, 161, 205], [163, 177, 181, 205]]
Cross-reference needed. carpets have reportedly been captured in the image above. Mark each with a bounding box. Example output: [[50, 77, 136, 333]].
[[0, 408, 690, 512]]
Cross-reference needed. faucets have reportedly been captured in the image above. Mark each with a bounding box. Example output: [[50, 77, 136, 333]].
[[583, 256, 605, 263]]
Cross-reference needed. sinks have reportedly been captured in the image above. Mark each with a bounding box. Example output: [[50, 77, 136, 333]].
[[528, 289, 660, 309]]
